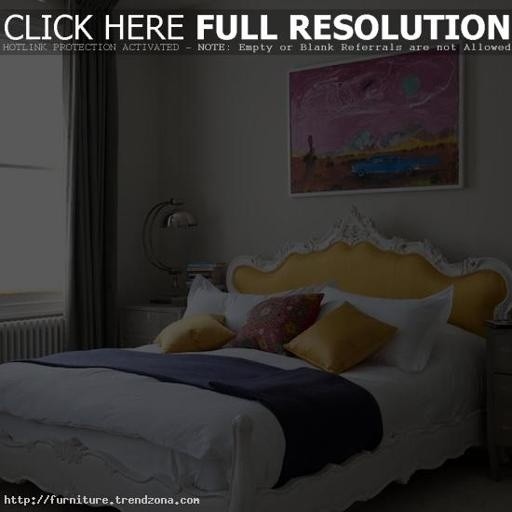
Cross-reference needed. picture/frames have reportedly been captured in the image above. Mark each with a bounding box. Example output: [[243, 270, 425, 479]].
[[286, 51, 464, 195]]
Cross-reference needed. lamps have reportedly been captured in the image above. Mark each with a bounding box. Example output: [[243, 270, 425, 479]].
[[142, 194, 198, 296]]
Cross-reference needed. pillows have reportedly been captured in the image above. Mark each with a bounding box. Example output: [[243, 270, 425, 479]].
[[286, 302, 402, 371], [234, 290, 324, 356], [183, 274, 308, 360], [153, 312, 231, 356], [320, 283, 455, 373]]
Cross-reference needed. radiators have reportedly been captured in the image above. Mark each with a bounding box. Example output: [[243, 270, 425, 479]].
[[1, 313, 67, 365]]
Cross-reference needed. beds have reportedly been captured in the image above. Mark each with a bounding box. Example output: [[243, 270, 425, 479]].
[[0, 204, 512, 512]]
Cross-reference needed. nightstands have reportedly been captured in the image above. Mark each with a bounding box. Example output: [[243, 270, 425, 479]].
[[118, 304, 182, 349], [486, 315, 512, 477]]
